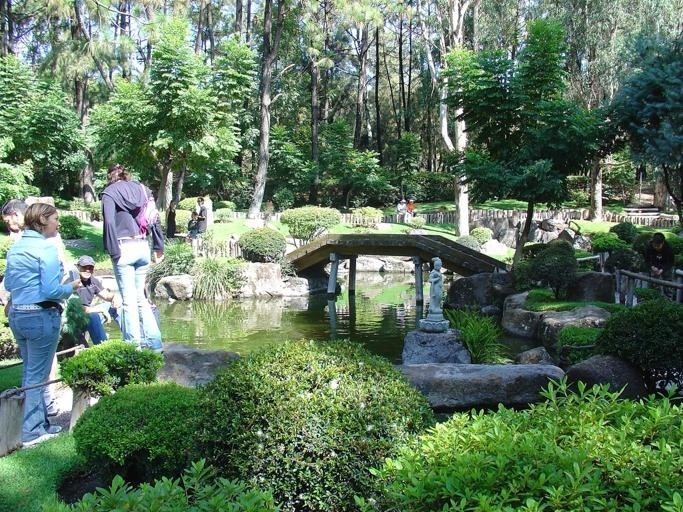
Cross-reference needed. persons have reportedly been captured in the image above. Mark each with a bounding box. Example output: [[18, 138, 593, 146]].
[[396, 199, 410, 223], [100, 162, 165, 354], [63, 253, 122, 346], [166, 199, 176, 238], [1, 197, 58, 418], [2, 202, 80, 445], [645, 232, 674, 297], [406, 199, 415, 213], [427, 256, 444, 313], [186, 210, 198, 238], [195, 197, 206, 231]]
